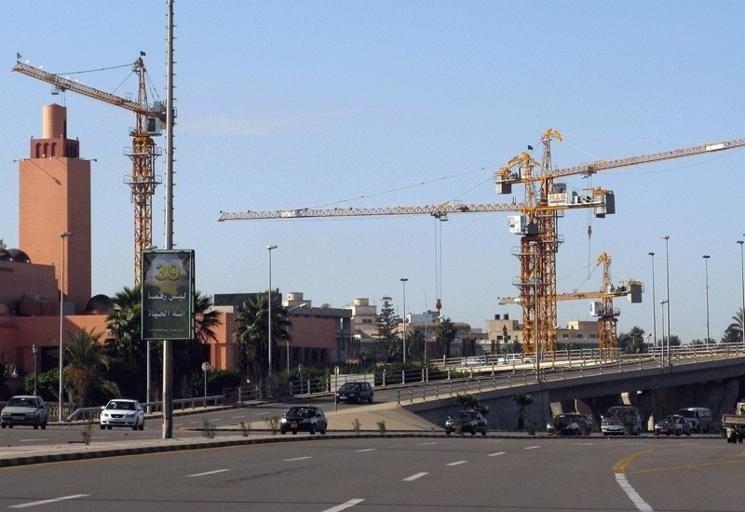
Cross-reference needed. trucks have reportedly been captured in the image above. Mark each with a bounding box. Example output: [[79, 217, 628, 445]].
[[722, 401, 745, 443]]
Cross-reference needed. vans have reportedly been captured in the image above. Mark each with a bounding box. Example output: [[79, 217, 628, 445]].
[[679, 407, 712, 433], [600, 405, 641, 435]]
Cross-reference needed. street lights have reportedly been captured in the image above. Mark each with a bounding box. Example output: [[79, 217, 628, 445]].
[[735, 240, 745, 343], [268, 244, 277, 399], [702, 255, 711, 353], [661, 235, 670, 365], [400, 279, 409, 363], [648, 252, 656, 346], [659, 299, 669, 368], [59, 232, 74, 421], [32, 343, 38, 395]]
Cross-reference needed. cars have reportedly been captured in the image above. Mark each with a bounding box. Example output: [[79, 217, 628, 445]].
[[0, 395, 49, 430], [461, 353, 535, 367], [446, 409, 488, 435], [99, 398, 144, 431], [336, 382, 375, 403], [654, 415, 691, 437], [279, 406, 327, 435], [545, 414, 594, 436]]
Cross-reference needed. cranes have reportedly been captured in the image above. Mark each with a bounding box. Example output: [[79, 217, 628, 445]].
[[492, 133, 744, 356], [221, 152, 617, 357], [494, 251, 645, 350], [12, 53, 166, 290]]
